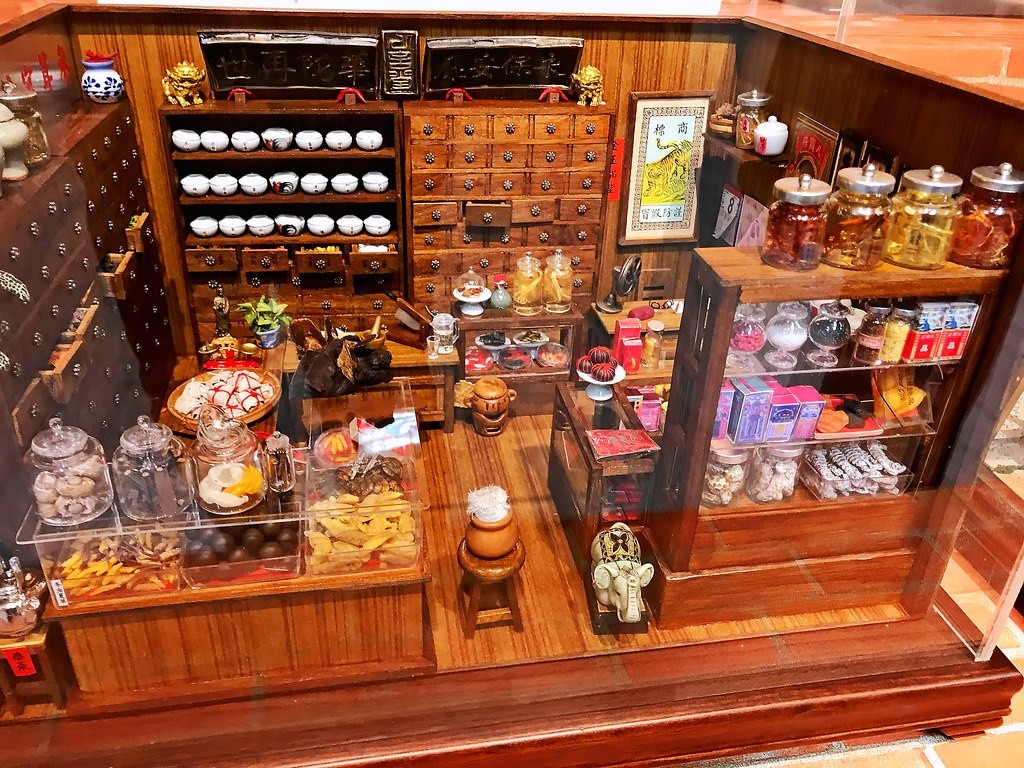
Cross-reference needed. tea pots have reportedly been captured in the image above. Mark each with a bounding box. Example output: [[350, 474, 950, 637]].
[[0, 586, 40, 637]]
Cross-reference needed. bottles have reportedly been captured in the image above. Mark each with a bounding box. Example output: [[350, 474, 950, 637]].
[[189, 406, 268, 516], [760, 174, 833, 272], [112, 414, 194, 521], [880, 164, 963, 271], [511, 248, 574, 316], [640, 320, 664, 369], [736, 89, 772, 150], [948, 162, 1024, 270], [24, 417, 115, 527], [0, 80, 52, 168], [853, 301, 916, 367], [820, 162, 897, 270], [743, 445, 805, 507], [265, 431, 296, 493], [700, 448, 750, 509]]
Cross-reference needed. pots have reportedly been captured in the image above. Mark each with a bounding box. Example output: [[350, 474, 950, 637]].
[[463, 377, 517, 417]]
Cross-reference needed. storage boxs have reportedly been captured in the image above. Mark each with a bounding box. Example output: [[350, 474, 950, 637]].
[[35, 448, 422, 608], [901, 300, 980, 364], [712, 367, 937, 504]]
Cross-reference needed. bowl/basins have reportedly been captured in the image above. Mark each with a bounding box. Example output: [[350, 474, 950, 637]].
[[172, 128, 392, 237], [465, 341, 570, 371], [753, 115, 788, 156]]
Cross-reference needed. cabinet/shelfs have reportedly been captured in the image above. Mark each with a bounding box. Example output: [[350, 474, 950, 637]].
[[158, 98, 407, 364], [645, 246, 1010, 573], [450, 301, 585, 417], [404, 94, 620, 337], [0, 99, 179, 569], [547, 380, 661, 579]]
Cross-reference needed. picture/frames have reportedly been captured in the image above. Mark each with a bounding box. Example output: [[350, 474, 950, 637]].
[[617, 89, 717, 246]]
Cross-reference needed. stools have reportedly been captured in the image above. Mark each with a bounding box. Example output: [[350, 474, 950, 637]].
[[0, 571, 70, 716], [456, 538, 526, 640]]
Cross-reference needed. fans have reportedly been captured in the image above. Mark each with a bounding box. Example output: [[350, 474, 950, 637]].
[[595, 255, 643, 315]]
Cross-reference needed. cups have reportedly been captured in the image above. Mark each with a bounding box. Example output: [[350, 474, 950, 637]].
[[429, 312, 461, 355], [426, 336, 440, 359]]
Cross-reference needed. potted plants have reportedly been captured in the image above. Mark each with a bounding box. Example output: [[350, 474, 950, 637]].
[[233, 295, 293, 350]]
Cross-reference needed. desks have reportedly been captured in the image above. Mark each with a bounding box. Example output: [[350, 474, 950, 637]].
[[284, 334, 460, 440], [589, 299, 685, 359], [42, 513, 433, 694]]
